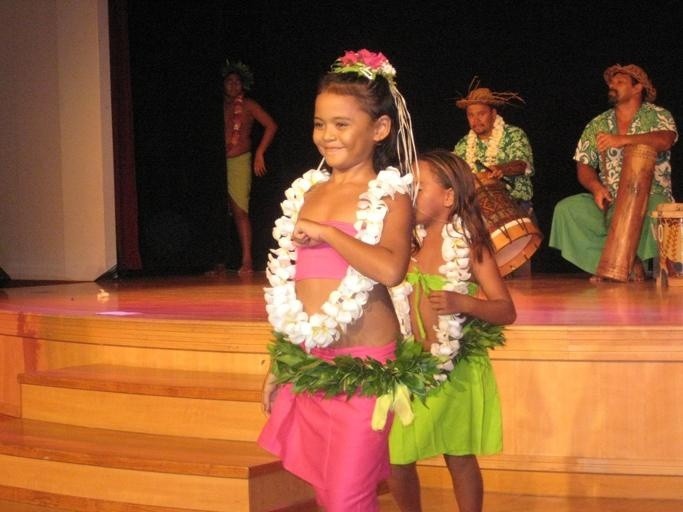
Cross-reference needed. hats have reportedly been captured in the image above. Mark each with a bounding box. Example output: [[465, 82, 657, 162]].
[[602, 64, 656, 103], [456, 87, 527, 110]]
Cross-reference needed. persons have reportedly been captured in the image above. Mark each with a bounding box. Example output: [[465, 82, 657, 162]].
[[386, 147, 517, 512], [449, 75, 535, 211], [548, 62, 678, 286], [224, 60, 278, 276], [257, 49, 420, 512]]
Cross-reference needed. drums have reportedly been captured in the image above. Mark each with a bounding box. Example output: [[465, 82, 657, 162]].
[[651, 203, 683, 288], [597, 144, 657, 281], [470, 173, 543, 276]]
